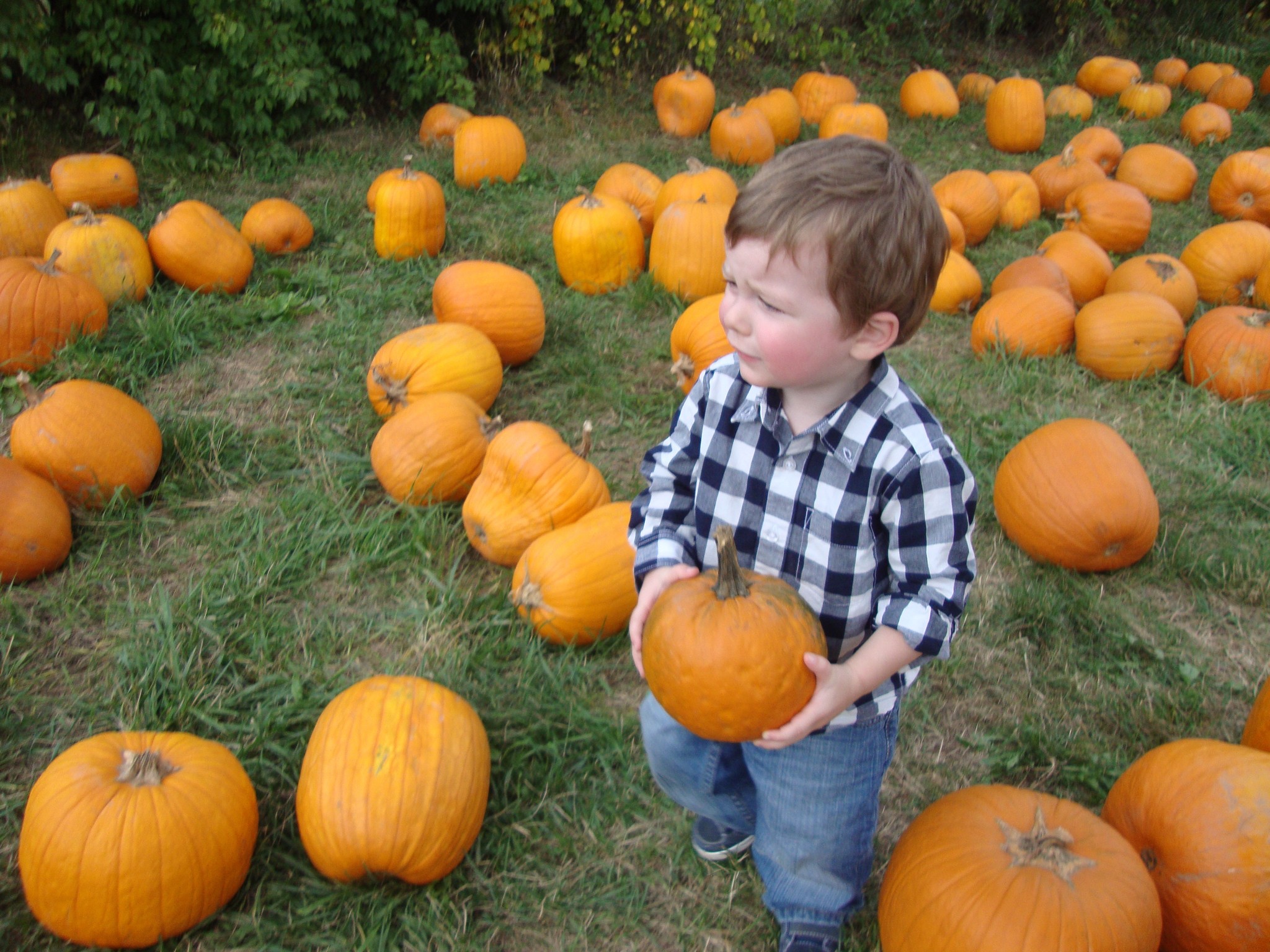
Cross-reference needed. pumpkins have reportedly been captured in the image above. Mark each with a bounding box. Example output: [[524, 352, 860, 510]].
[[296, 674, 491, 882], [511, 500, 639, 646], [420, 102, 474, 150], [148, 199, 255, 297], [373, 154, 446, 261], [653, 60, 887, 165], [367, 167, 428, 212], [432, 259, 545, 369], [899, 49, 1270, 402], [1239, 674, 1270, 752], [18, 731, 258, 948], [11, 371, 162, 512], [241, 196, 313, 256], [642, 526, 828, 742], [553, 155, 735, 396], [50, 153, 139, 212], [992, 419, 1159, 572], [0, 456, 73, 583], [461, 418, 610, 565], [370, 392, 504, 508], [44, 201, 155, 310], [876, 784, 1163, 952], [0, 174, 70, 258], [367, 322, 503, 420], [1101, 737, 1270, 952], [0, 249, 109, 377], [452, 114, 527, 187]]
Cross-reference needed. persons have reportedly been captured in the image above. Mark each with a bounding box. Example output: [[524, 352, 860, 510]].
[[627, 135, 979, 952]]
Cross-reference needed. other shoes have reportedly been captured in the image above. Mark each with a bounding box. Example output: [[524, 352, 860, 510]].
[[692, 816, 754, 860], [779, 920, 841, 952]]
